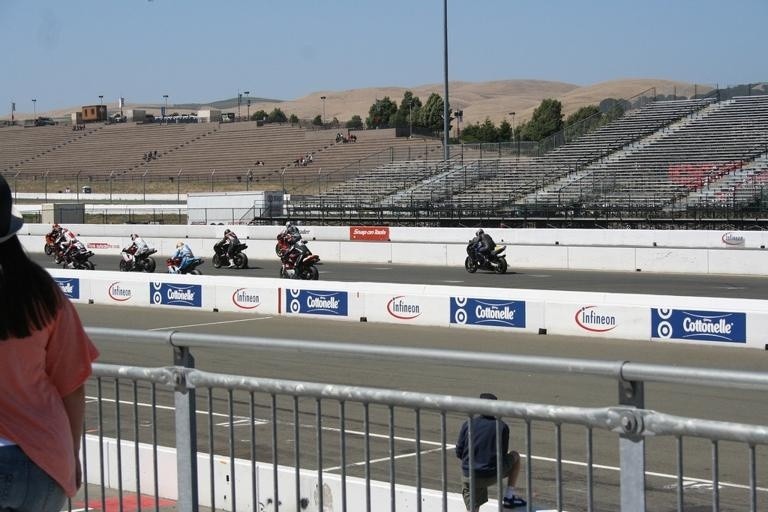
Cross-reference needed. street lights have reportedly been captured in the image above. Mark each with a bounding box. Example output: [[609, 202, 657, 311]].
[[509, 111, 515, 149], [408, 97, 412, 136], [98, 95, 103, 122], [320, 96, 326, 125], [163, 95, 168, 125], [454, 108, 463, 146], [244, 91, 251, 121], [32, 99, 36, 124]]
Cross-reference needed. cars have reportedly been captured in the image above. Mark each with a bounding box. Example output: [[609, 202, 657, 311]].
[[109, 113, 128, 122], [169, 111, 197, 116]]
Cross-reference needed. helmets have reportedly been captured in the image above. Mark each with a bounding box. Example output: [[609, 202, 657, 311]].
[[131, 234, 138, 240], [475, 229, 483, 236], [176, 242, 184, 248], [224, 229, 230, 235], [286, 221, 294, 229]]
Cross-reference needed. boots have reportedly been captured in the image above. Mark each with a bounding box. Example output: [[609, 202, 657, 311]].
[[228, 259, 236, 267]]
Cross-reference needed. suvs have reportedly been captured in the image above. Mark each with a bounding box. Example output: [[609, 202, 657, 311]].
[[37, 117, 58, 126]]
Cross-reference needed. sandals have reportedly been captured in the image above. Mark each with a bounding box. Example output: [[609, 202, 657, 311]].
[[503, 495, 527, 508]]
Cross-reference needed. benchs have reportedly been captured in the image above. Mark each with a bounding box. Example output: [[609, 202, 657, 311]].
[[313, 96, 768, 208]]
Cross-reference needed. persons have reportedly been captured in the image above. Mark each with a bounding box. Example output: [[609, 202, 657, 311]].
[[281, 220, 299, 249], [2, 173, 101, 510], [284, 237, 310, 275], [126, 233, 150, 271], [220, 228, 243, 268], [54, 227, 78, 251], [64, 238, 88, 269], [49, 223, 63, 239], [468, 229, 497, 269], [170, 241, 195, 273], [456, 391, 531, 510]]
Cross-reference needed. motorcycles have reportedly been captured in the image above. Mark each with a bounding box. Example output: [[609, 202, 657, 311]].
[[44, 231, 66, 255], [275, 228, 320, 280], [59, 243, 97, 270], [463, 238, 509, 274], [166, 254, 204, 275], [119, 246, 158, 272], [212, 239, 248, 270]]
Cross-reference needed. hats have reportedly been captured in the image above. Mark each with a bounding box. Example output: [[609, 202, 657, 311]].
[[1, 174, 24, 243]]
